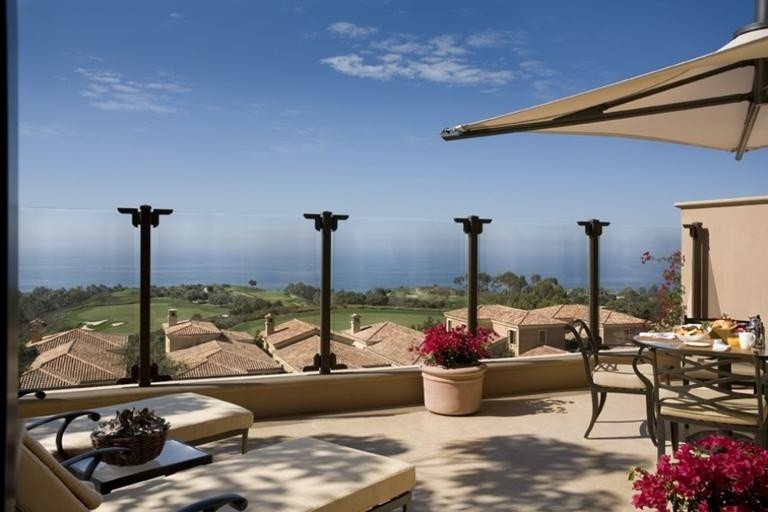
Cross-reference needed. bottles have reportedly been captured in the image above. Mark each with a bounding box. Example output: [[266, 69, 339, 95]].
[[746, 316, 765, 350]]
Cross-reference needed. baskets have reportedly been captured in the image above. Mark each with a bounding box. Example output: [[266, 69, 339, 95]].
[[90, 429, 167, 466]]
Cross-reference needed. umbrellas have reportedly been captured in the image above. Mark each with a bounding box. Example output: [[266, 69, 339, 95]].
[[438, 1, 767, 165]]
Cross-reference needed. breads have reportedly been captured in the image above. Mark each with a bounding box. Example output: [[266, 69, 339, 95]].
[[709, 319, 733, 329]]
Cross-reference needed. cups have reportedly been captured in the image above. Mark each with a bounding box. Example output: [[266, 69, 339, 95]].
[[739, 332, 756, 350], [727, 334, 740, 349]]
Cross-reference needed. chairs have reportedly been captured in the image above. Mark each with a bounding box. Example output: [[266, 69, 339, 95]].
[[561, 315, 767, 469]]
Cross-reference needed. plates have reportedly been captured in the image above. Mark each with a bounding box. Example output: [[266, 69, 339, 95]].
[[684, 341, 711, 347]]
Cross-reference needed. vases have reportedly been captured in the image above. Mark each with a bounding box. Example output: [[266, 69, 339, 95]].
[[420, 366, 487, 416]]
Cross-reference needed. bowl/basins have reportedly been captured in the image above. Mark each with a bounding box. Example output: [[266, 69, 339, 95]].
[[713, 328, 738, 340], [673, 323, 712, 342]]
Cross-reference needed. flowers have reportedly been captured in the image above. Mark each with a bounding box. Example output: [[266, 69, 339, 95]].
[[406, 315, 500, 370], [624, 430, 767, 512]]
